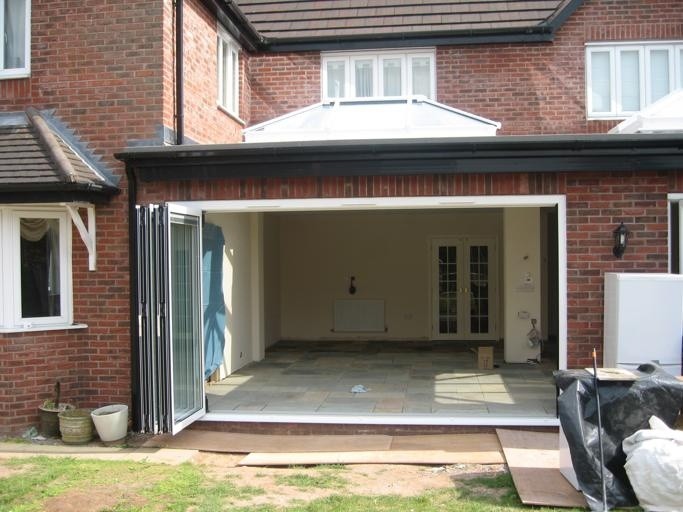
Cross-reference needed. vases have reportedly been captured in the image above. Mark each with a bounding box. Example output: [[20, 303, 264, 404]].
[[37, 402, 129, 445]]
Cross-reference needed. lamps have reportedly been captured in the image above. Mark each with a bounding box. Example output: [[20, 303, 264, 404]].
[[613, 219, 628, 258]]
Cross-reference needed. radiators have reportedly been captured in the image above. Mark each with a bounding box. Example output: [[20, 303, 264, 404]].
[[332, 299, 387, 332]]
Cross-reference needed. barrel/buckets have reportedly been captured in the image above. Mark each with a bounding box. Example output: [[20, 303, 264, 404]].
[[90, 404, 129, 441], [37, 402, 78, 437], [56, 407, 95, 444]]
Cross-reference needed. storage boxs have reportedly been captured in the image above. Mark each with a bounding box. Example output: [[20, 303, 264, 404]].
[[471, 344, 494, 370]]
